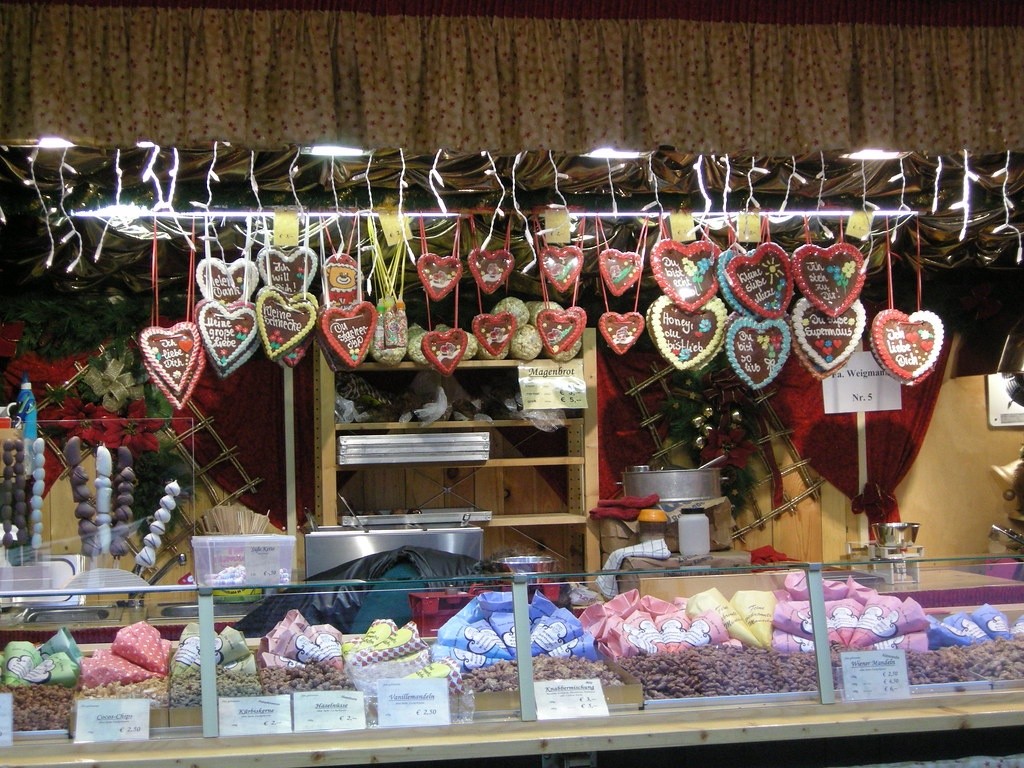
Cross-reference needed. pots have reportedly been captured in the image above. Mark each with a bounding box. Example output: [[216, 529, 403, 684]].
[[614, 468, 729, 512]]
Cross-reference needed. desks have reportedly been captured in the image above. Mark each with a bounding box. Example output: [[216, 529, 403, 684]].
[[861, 568, 1024, 607]]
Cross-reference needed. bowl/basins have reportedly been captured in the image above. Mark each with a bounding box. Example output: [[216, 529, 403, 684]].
[[492, 555, 559, 584], [871, 523, 920, 547]]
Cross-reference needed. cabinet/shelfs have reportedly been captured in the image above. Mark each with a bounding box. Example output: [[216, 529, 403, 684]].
[[314, 329, 599, 594]]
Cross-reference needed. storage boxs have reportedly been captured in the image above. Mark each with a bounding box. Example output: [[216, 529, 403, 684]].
[[68, 638, 644, 741], [190, 533, 296, 596], [599, 495, 736, 554]]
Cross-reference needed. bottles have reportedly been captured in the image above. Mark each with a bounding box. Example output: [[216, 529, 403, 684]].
[[17, 371, 38, 472], [0, 417, 18, 477]]
